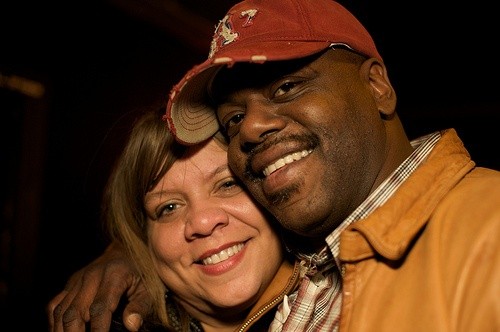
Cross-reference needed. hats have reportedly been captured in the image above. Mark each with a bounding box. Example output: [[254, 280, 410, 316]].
[[166, 0, 384, 145]]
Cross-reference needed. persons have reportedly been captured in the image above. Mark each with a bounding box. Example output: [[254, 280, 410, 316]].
[[84, 98, 302, 332], [49, 0, 500, 332]]
